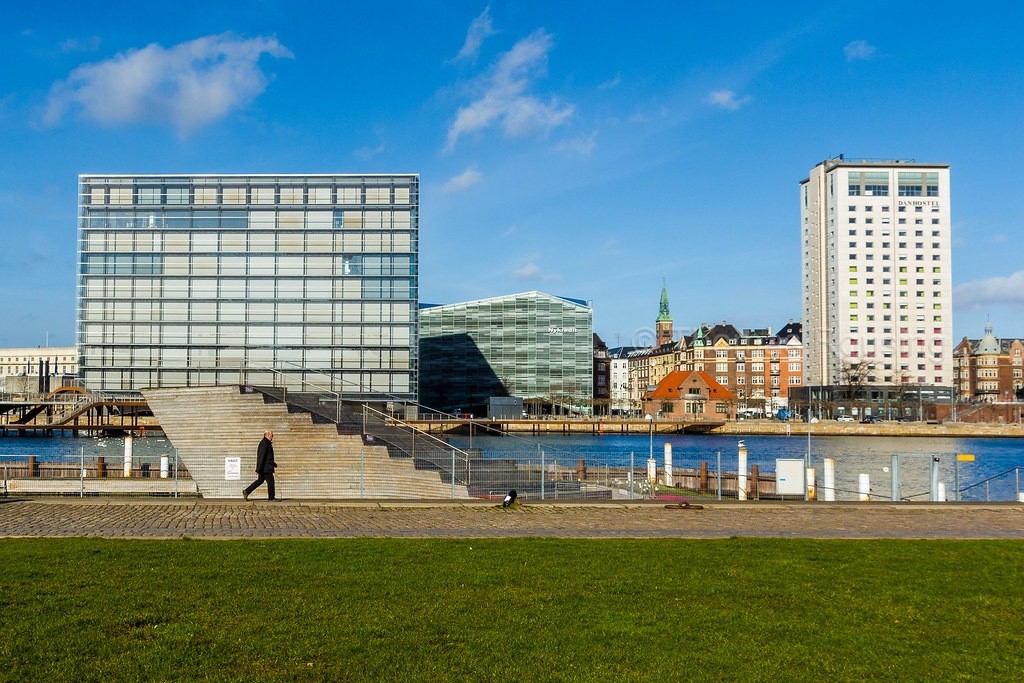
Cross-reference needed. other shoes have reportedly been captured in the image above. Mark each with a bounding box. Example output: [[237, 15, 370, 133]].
[[243, 490, 248, 502], [268, 498, 281, 502]]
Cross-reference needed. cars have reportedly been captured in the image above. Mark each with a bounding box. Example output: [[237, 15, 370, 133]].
[[838, 415, 854, 422], [860, 415, 883, 425]]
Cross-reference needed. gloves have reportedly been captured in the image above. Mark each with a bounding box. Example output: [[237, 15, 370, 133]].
[[273, 462, 278, 468]]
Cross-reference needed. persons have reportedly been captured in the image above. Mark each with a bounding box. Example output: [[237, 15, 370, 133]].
[[244, 430, 277, 501]]
